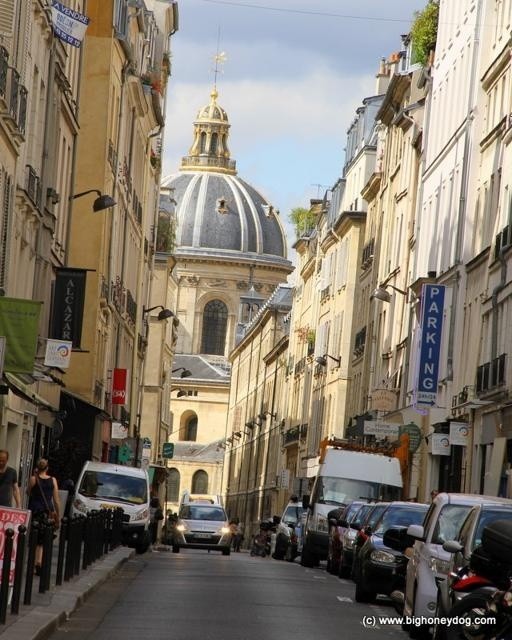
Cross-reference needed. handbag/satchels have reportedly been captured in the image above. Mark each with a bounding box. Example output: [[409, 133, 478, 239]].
[[47, 511, 59, 532]]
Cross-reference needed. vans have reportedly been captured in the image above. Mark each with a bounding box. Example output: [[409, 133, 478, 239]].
[[62, 458, 161, 556]]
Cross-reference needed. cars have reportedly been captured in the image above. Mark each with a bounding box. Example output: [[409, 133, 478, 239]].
[[173, 504, 235, 558], [249, 435, 511, 640], [162, 515, 179, 546]]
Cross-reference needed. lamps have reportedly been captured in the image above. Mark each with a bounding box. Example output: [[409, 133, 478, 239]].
[[170, 387, 187, 397], [370, 284, 409, 303], [234, 411, 277, 438], [171, 367, 192, 378], [142, 305, 174, 321], [71, 188, 117, 212], [313, 352, 342, 369]]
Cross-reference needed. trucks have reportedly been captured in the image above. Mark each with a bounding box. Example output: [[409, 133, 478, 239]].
[[180, 493, 224, 509]]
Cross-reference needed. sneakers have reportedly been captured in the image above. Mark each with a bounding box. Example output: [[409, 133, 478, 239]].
[[34, 563, 41, 576]]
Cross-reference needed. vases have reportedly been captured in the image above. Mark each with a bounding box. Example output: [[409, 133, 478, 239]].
[[308, 348, 314, 355]]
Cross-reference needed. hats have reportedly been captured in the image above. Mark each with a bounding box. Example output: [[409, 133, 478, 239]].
[[82, 475, 103, 489]]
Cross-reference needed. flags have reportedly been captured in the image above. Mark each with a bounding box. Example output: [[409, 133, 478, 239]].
[[50, 267, 87, 350], [112, 368, 127, 407], [49, 2, 92, 49], [110, 420, 129, 440], [41, 337, 74, 370], [448, 421, 470, 446], [431, 432, 452, 456], [0, 295, 44, 378]]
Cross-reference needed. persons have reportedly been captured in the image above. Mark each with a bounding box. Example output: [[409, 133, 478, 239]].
[[423, 490, 440, 505], [162, 513, 179, 545], [27, 458, 61, 577], [149, 496, 163, 545], [271, 523, 298, 560], [253, 530, 267, 556], [285, 525, 299, 562], [229, 515, 246, 553], [0, 449, 24, 511]]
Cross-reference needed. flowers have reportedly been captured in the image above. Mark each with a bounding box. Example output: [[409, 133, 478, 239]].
[[294, 326, 315, 348]]
